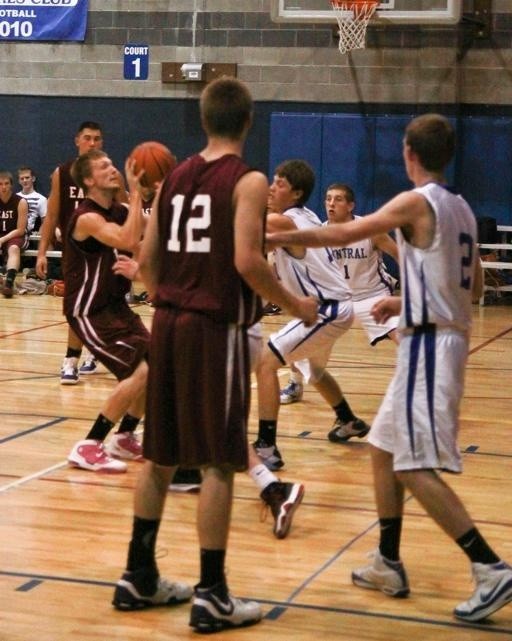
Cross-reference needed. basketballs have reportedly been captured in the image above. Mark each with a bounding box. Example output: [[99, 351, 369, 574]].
[[129, 141, 176, 188]]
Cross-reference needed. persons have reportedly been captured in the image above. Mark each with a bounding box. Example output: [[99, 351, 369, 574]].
[[35, 121, 125, 385], [138, 185, 159, 217], [0, 171, 30, 298], [111, 239, 303, 541], [12, 166, 49, 234], [249, 159, 372, 471], [114, 75, 263, 634], [263, 112, 512, 623], [58, 151, 180, 473], [260, 302, 278, 316], [276, 182, 399, 406]]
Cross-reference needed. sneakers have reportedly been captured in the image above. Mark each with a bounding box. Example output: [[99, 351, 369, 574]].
[[279, 380, 303, 405], [351, 560, 412, 600], [59, 360, 81, 388], [452, 560, 512, 622], [81, 353, 101, 375], [168, 466, 202, 494], [188, 582, 263, 634], [2, 286, 14, 300], [256, 479, 301, 536], [66, 437, 129, 476], [110, 570, 194, 612], [253, 439, 285, 472], [328, 416, 370, 445], [101, 428, 145, 458], [133, 290, 156, 308], [263, 304, 282, 316]]
